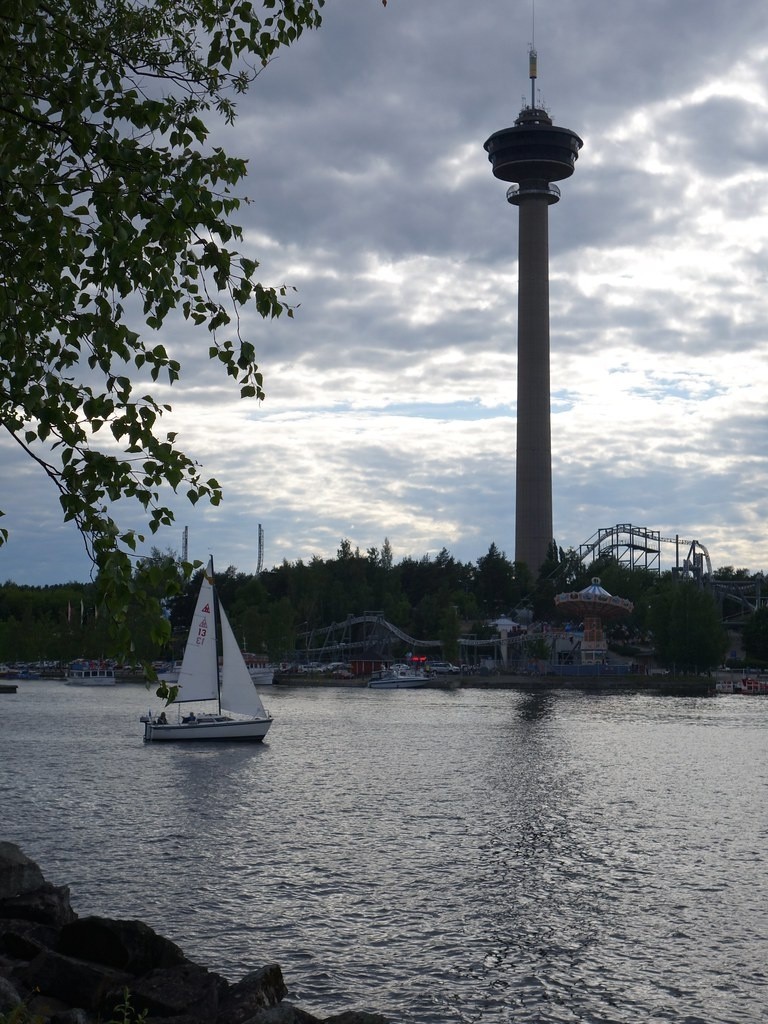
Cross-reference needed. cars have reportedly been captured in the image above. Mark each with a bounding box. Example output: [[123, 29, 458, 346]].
[[278, 661, 352, 673], [0, 656, 183, 672], [389, 664, 410, 671], [332, 670, 355, 680]]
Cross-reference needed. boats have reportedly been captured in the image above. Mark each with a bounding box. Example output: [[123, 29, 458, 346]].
[[715, 676, 768, 697], [368, 670, 430, 688], [64, 658, 116, 685], [150, 632, 275, 685], [0, 662, 41, 682]]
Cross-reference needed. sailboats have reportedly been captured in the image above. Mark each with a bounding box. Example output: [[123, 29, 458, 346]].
[[138, 554, 274, 743]]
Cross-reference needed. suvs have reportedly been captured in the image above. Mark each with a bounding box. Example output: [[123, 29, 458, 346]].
[[429, 662, 460, 677]]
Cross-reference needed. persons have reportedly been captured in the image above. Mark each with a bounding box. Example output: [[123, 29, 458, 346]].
[[157, 712, 167, 725], [464, 623, 645, 678], [182, 712, 195, 723]]
